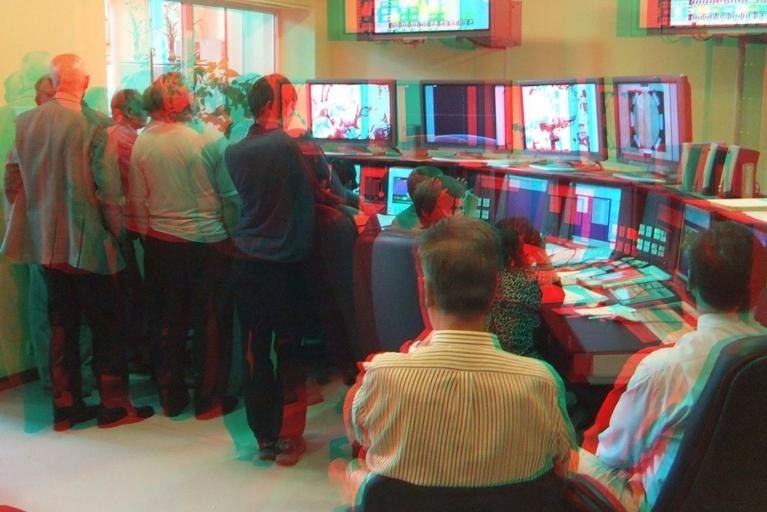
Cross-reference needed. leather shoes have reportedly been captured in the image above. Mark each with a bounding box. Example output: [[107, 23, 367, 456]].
[[54, 404, 97, 430], [196, 397, 237, 419], [98, 406, 154, 428]]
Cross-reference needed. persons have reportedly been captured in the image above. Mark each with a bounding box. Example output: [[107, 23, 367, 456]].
[[569, 216, 766, 512], [0, 51, 571, 468], [342, 214, 584, 505]]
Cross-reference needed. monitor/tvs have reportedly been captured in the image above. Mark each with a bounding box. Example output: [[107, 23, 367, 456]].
[[517, 78, 608, 172], [353, 164, 360, 196], [612, 77, 693, 188], [674, 203, 714, 288], [558, 181, 636, 263], [387, 167, 415, 217], [496, 174, 565, 243], [307, 78, 401, 159], [421, 80, 513, 153], [355, 1, 524, 49], [638, 1, 767, 40]]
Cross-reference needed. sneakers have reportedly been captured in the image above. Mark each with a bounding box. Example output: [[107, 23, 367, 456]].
[[258, 436, 304, 466]]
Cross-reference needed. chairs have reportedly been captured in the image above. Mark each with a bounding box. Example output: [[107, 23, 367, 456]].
[[353, 465, 601, 511], [569, 335, 767, 512]]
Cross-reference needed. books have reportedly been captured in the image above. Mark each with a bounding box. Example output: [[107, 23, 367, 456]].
[[544, 235, 679, 324]]
